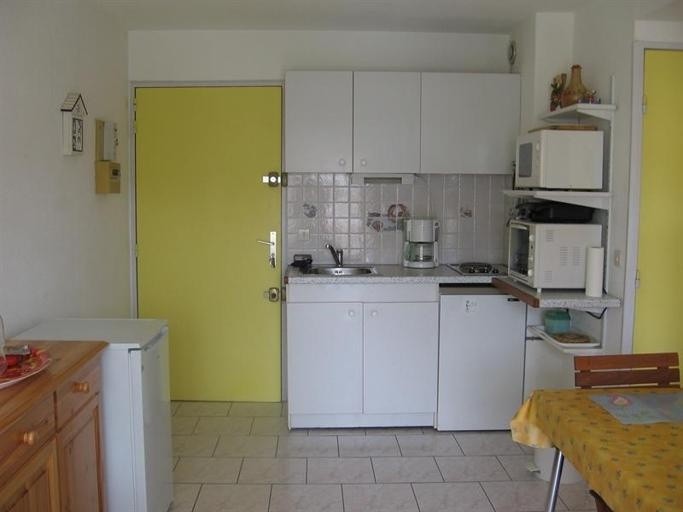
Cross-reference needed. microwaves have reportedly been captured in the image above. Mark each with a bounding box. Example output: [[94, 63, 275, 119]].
[[515, 129, 604, 190]]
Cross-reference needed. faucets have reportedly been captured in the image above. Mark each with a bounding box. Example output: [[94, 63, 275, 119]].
[[325, 244, 342, 267]]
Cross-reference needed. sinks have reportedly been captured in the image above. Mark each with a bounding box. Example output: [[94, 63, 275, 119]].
[[299, 266, 378, 277]]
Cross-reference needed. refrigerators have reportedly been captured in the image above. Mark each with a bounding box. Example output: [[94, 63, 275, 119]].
[[12, 319, 174, 512]]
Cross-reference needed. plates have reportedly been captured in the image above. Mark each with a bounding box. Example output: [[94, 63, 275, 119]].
[[0, 344, 52, 389], [533, 325, 600, 348]]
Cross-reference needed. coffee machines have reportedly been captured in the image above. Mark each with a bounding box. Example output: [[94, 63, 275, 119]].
[[402, 219, 441, 269]]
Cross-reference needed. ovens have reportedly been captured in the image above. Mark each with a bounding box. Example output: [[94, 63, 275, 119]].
[[507, 219, 602, 293]]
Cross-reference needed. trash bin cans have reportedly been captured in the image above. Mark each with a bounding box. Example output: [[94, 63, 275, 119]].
[[526, 444, 582, 484]]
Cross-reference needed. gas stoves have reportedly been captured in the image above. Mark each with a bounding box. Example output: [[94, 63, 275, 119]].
[[447, 260, 509, 276]]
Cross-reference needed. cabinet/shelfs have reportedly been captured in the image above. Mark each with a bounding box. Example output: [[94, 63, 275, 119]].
[[491, 102, 621, 356], [283, 282, 439, 431], [283, 68, 419, 175], [437, 291, 577, 433], [420, 71, 523, 175], [1, 338, 109, 512]]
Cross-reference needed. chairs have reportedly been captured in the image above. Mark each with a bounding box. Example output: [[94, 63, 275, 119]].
[[572, 348, 682, 512]]
